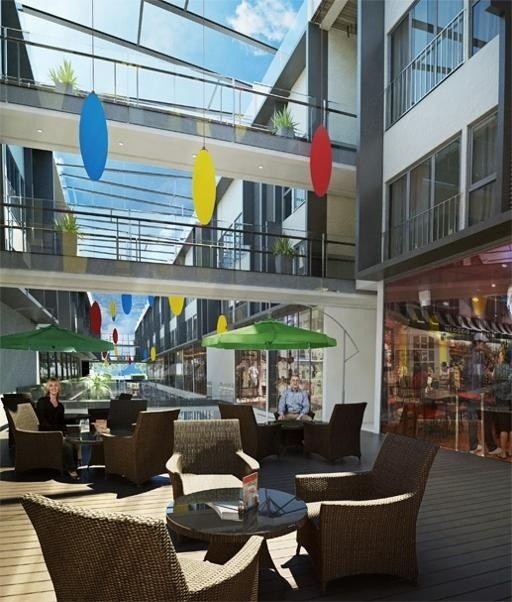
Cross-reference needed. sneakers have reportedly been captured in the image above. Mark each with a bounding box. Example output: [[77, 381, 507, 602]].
[[470, 444, 512, 459]]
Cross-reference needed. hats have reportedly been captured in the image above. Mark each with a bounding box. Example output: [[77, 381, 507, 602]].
[[473, 332, 490, 342]]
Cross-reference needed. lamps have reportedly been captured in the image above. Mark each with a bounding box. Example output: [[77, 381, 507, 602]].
[[308, 1, 333, 197], [193, 1, 216, 225], [90, 294, 227, 366], [79, 0, 108, 181]]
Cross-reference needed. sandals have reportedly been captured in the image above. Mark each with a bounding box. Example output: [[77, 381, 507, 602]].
[[66, 469, 80, 480]]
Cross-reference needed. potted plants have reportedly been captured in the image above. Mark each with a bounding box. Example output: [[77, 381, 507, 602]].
[[269, 105, 302, 138], [271, 236, 297, 274], [49, 58, 78, 95], [54, 212, 83, 256]]
